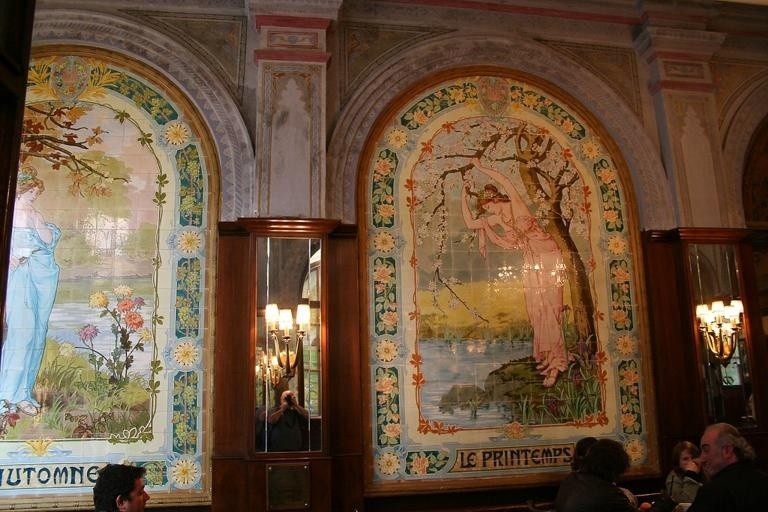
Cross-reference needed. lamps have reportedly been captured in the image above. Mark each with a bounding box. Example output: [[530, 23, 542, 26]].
[[694, 298, 745, 365], [266, 302, 313, 380]]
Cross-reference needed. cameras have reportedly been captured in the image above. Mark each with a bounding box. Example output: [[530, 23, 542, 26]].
[[285, 395, 294, 406]]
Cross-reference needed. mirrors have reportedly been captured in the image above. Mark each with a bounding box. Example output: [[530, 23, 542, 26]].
[[686, 240, 759, 430], [254, 234, 324, 454]]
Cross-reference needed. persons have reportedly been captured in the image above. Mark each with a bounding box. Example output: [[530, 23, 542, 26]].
[[663, 440, 709, 504], [458, 155, 573, 389], [92, 462, 153, 510], [683, 423, 767, 512], [2, 162, 64, 423]]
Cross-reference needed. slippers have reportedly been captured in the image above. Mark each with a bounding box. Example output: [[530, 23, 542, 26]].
[[536, 362, 561, 388], [15, 399, 39, 418]]
